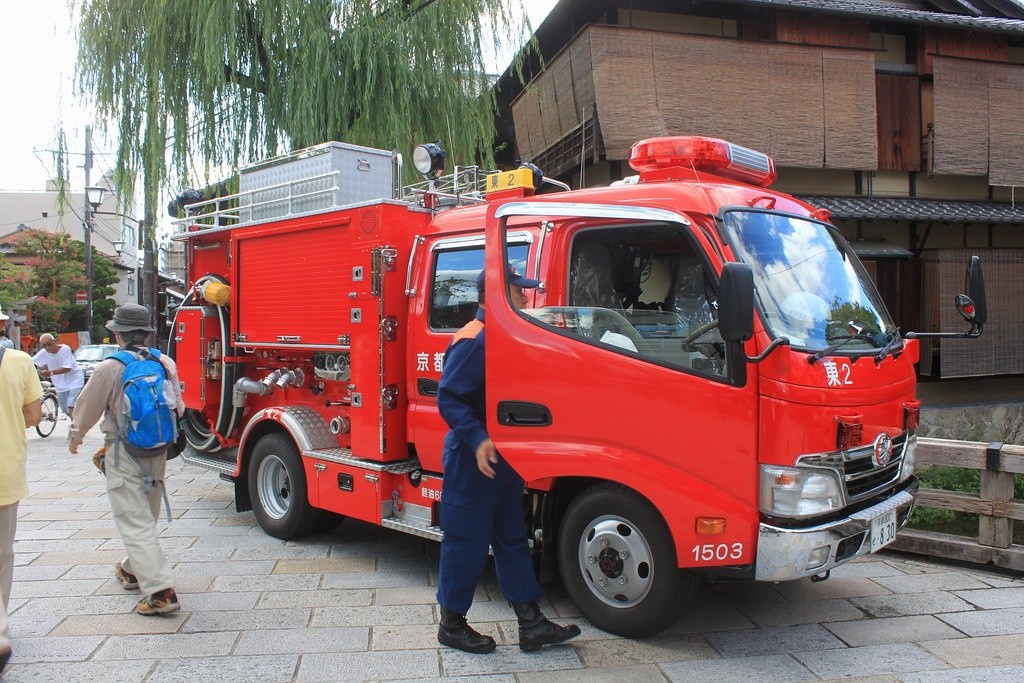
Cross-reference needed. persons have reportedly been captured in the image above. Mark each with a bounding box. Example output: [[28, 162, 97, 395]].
[[32, 332, 83, 422], [436, 263, 582, 654], [67, 303, 183, 613], [0, 305, 45, 656]]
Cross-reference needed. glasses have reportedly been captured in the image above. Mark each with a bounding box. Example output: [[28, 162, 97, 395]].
[[41, 341, 52, 348]]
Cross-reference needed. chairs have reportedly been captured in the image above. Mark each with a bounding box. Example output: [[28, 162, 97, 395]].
[[572, 241, 621, 334], [669, 258, 716, 340]]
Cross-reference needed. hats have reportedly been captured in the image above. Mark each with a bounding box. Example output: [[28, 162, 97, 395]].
[[477, 262, 541, 288], [0, 311, 10, 320], [105, 303, 156, 333]]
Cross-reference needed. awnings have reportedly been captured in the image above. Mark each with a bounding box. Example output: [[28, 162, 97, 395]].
[[846, 240, 914, 259]]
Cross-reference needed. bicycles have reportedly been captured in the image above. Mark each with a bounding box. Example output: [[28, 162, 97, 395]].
[[32, 364, 67, 438]]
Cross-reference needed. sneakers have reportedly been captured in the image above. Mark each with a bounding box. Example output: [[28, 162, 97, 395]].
[[115, 562, 139, 590], [136, 588, 181, 616]]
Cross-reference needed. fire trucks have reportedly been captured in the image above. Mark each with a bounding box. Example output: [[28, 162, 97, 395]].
[[168, 133, 987, 634]]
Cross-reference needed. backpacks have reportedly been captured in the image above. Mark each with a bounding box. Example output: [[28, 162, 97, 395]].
[[102, 347, 180, 458]]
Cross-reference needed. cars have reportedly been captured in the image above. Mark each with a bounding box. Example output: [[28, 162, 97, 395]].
[[73, 344, 121, 383]]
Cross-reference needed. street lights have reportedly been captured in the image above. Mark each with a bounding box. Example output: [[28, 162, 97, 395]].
[[83, 184, 144, 306], [84, 239, 122, 345]]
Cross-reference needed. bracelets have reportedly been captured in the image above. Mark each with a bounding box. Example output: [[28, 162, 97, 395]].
[[50, 369, 54, 375]]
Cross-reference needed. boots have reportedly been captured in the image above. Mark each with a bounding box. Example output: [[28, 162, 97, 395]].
[[438, 605, 497, 654], [508, 600, 581, 652]]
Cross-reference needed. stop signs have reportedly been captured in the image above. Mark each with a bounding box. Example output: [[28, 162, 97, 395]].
[[76, 290, 88, 301]]
